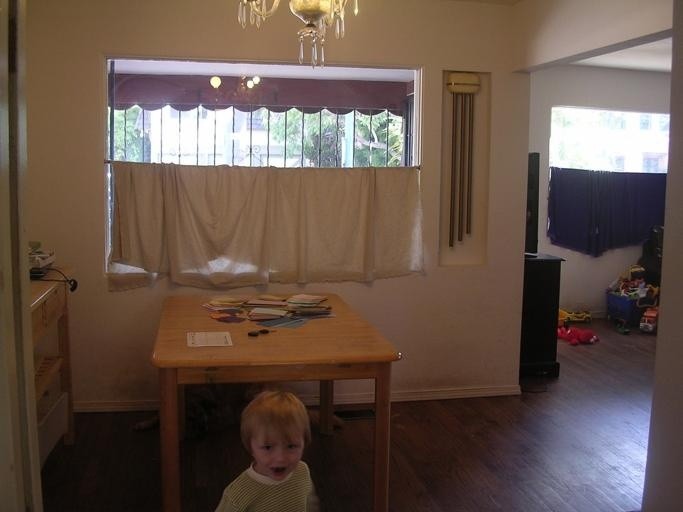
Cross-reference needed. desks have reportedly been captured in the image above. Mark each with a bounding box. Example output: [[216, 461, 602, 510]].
[[152, 294, 401, 512], [520, 259, 567, 381]]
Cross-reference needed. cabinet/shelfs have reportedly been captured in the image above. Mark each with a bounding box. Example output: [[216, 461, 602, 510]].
[[28, 272, 75, 470]]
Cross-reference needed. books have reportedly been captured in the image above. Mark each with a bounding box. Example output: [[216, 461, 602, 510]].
[[201, 292, 336, 323]]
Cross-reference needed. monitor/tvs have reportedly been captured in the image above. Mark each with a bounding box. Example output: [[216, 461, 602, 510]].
[[526, 153, 540, 257]]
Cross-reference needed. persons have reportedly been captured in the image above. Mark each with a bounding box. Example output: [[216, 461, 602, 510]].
[[209, 388, 320, 512]]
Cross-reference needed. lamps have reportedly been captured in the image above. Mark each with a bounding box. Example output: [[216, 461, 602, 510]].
[[209, 75, 263, 90], [237, 0, 359, 69]]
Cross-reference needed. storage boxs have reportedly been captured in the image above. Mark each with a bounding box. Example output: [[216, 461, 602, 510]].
[[607, 292, 652, 322]]
